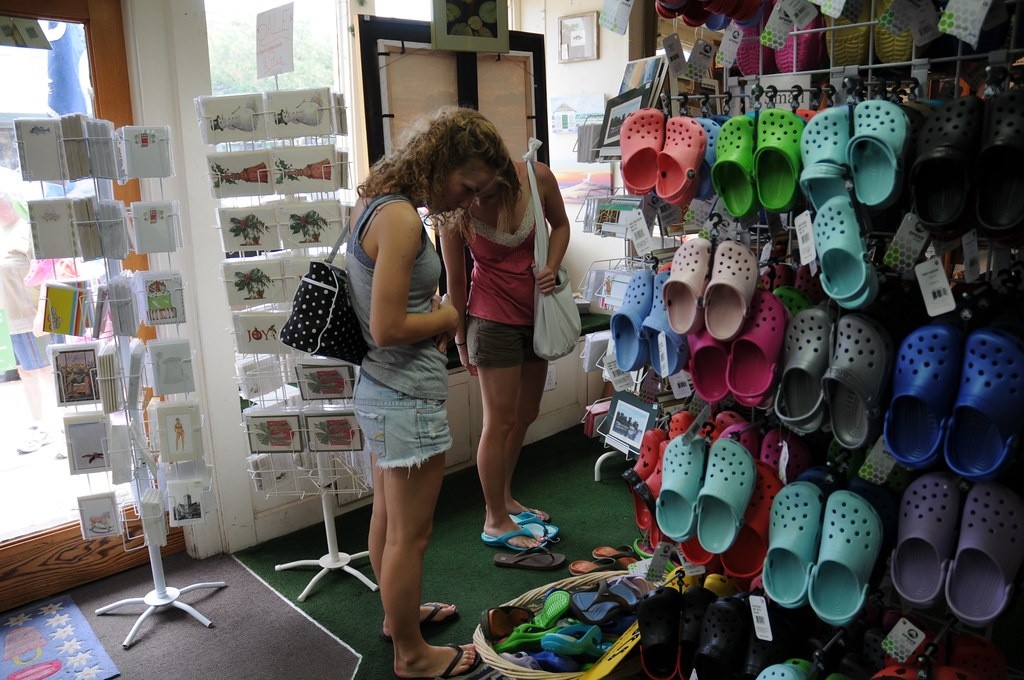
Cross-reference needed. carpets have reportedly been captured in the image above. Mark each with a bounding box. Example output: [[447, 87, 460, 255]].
[[0, 593, 122, 680]]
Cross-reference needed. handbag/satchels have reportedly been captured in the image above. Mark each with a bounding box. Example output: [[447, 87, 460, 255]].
[[521, 136, 582, 360], [277, 217, 365, 368]]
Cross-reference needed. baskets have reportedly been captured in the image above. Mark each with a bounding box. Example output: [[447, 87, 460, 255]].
[[472, 571, 666, 680]]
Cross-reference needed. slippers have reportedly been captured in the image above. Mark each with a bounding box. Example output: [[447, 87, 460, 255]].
[[381, 602, 458, 638], [493, 546, 566, 569], [481, 524, 548, 550], [395, 643, 482, 680], [480, 0, 1024, 680], [509, 512, 559, 539]]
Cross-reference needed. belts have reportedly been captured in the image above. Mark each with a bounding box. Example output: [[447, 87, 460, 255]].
[[359, 367, 392, 390]]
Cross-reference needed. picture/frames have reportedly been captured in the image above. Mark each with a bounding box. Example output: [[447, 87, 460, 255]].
[[559, 10, 600, 64], [430, 0, 510, 55]]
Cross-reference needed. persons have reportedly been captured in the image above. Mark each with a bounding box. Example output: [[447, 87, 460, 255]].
[[439, 116, 570, 551], [0, 195, 68, 460], [346, 108, 509, 680]]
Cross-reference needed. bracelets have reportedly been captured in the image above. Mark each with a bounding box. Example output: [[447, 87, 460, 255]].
[[455, 342, 465, 346]]
